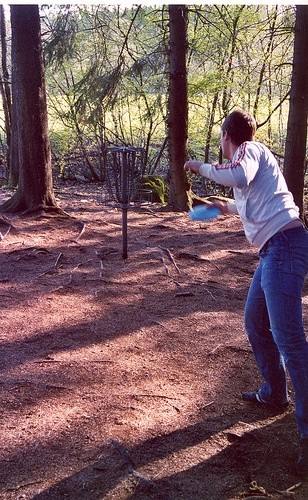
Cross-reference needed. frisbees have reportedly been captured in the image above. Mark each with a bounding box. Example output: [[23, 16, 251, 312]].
[[188, 204, 221, 221]]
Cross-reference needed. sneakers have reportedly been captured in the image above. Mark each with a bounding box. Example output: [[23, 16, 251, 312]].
[[241, 392, 289, 407], [296, 439, 308, 473]]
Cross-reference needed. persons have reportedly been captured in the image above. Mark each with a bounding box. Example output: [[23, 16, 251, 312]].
[[183, 109, 308, 474]]
